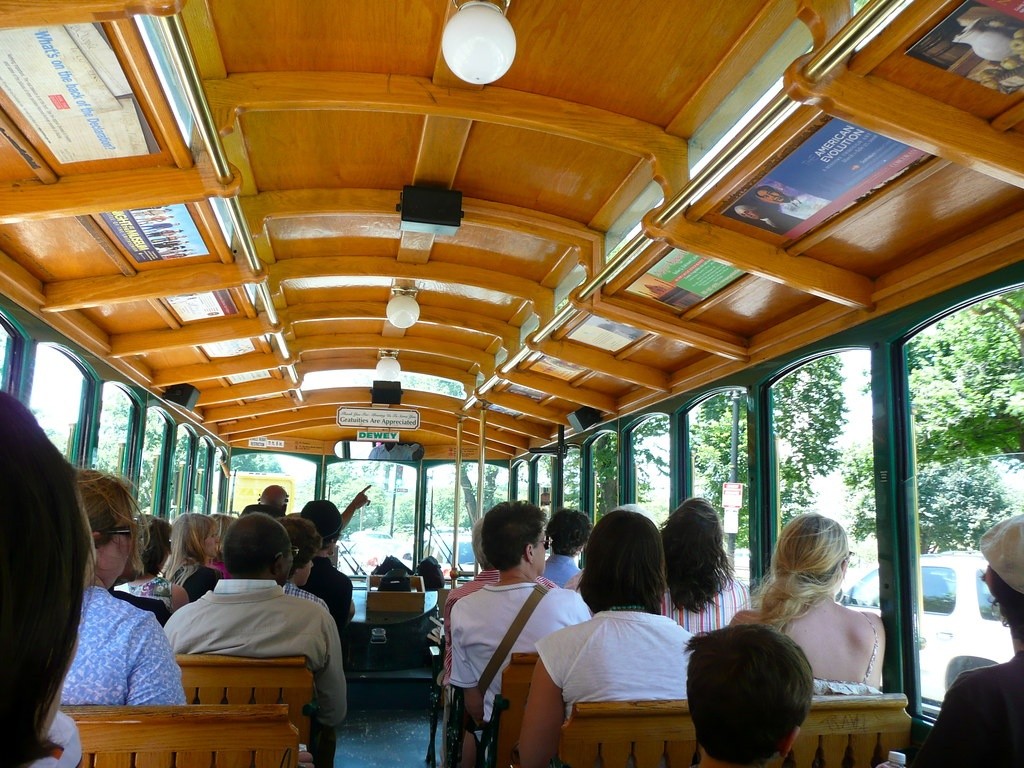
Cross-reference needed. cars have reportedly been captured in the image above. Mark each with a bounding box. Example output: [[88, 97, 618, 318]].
[[335, 524, 482, 580]]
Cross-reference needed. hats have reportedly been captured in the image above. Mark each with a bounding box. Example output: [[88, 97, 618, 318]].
[[980, 514, 1024, 595], [301, 499, 343, 540]]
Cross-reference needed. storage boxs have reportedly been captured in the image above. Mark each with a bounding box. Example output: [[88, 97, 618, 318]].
[[366, 575, 425, 612]]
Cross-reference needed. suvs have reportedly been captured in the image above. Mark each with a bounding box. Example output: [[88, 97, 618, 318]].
[[836, 550, 1015, 724]]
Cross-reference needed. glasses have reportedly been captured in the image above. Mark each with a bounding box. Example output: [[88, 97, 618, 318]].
[[539, 539, 551, 549], [283, 546, 299, 557]]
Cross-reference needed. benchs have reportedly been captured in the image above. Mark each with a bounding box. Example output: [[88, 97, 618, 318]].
[[38, 653, 314, 768], [454, 651, 912, 768]]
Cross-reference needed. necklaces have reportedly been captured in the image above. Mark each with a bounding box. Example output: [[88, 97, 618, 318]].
[[609, 604, 647, 613]]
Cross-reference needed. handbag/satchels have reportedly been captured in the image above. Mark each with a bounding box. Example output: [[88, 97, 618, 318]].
[[373, 553, 445, 592]]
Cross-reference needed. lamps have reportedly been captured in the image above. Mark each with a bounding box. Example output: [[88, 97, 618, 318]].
[[441, 0, 517, 84], [370, 380, 403, 405], [376, 351, 401, 381], [396, 185, 465, 238], [386, 286, 421, 328]]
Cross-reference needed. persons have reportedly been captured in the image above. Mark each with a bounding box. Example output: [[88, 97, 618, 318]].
[[62, 466, 188, 707], [730, 514, 885, 696], [443, 502, 695, 768], [105, 483, 374, 628], [162, 512, 347, 768], [684, 623, 815, 768], [660, 498, 751, 636], [0, 392, 102, 768], [909, 510, 1024, 768]]
